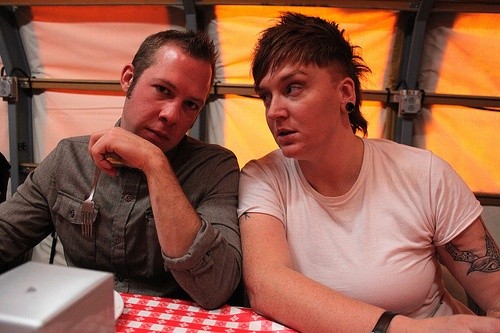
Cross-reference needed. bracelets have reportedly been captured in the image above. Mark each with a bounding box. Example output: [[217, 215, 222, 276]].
[[372, 310, 400, 332]]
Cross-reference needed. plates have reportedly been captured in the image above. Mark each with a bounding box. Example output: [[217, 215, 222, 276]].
[[113, 291, 124, 320]]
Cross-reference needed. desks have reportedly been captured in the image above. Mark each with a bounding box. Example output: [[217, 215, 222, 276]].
[[116, 288, 299, 333]]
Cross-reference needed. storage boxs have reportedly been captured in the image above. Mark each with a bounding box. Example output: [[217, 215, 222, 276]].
[[0, 262, 116, 332]]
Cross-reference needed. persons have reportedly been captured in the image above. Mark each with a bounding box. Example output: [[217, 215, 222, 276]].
[[0, 25, 242, 309], [238, 5, 500, 333]]
